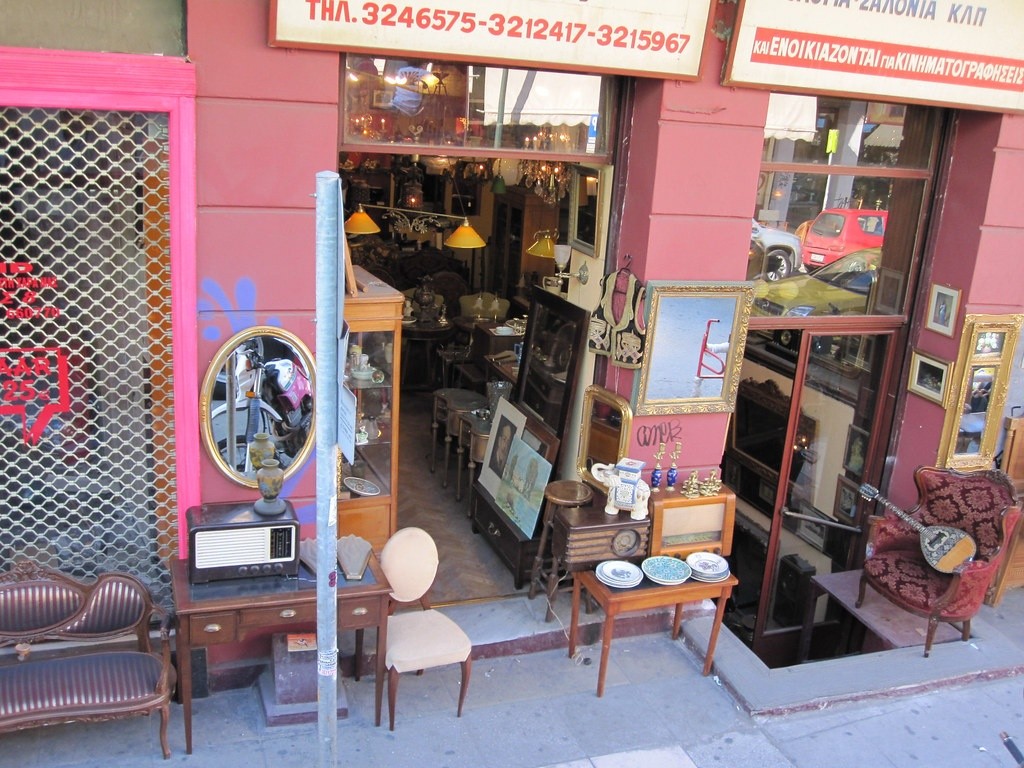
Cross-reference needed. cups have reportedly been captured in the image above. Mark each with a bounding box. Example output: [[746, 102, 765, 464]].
[[542, 276, 561, 295], [344, 354, 357, 381], [350, 460, 366, 480]]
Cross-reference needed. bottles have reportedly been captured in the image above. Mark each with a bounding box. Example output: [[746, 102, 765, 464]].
[[257, 459, 284, 500], [249, 433, 275, 470]]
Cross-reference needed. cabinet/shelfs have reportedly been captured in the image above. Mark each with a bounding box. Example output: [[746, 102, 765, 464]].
[[490, 185, 561, 318], [470, 484, 565, 590], [337, 267, 405, 560]]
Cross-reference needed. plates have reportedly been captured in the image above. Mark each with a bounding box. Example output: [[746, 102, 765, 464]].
[[506, 319, 528, 330], [344, 476, 380, 497], [642, 556, 693, 586], [401, 316, 417, 324], [594, 561, 644, 588], [684, 552, 731, 583]]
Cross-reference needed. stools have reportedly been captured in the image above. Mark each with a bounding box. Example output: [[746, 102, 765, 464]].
[[528, 480, 594, 622]]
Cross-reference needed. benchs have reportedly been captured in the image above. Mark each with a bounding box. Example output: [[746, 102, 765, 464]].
[[0, 561, 178, 759]]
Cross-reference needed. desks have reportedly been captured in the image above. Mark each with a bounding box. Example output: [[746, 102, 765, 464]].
[[430, 387, 493, 503], [170, 548, 394, 755], [401, 317, 453, 395], [570, 570, 740, 699]]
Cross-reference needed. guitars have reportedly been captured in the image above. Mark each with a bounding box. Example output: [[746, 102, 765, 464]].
[[857, 480, 977, 573]]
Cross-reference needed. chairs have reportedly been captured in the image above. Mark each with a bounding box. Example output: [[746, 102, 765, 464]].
[[348, 233, 511, 318], [380, 526, 471, 731], [854, 466, 1024, 658]]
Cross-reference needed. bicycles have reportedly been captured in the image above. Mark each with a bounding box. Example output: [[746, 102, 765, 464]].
[[203, 338, 287, 481]]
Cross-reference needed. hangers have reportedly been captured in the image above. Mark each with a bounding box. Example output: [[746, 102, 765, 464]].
[[599, 254, 646, 300]]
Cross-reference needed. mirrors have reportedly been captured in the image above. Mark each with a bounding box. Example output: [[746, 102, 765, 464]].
[[727, 376, 820, 508], [199, 326, 317, 490], [576, 386, 633, 495], [516, 285, 591, 483], [631, 279, 755, 417], [566, 163, 605, 258]]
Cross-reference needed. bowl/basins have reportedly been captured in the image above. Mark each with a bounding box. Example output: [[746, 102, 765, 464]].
[[351, 367, 377, 381]]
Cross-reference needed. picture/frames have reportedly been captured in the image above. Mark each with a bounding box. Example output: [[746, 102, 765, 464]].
[[925, 282, 962, 339], [906, 346, 954, 409], [842, 423, 870, 479], [758, 479, 777, 506], [795, 501, 831, 552], [833, 472, 863, 528], [725, 458, 741, 494], [369, 89, 395, 109], [936, 314, 1023, 471]]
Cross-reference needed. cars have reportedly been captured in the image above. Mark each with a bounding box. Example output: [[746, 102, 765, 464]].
[[802, 208, 890, 273], [747, 242, 887, 364], [751, 217, 804, 283]]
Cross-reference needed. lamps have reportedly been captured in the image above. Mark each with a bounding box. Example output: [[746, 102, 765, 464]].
[[340, 62, 578, 258], [470, 291, 484, 322], [489, 291, 502, 323], [553, 244, 589, 285]]
